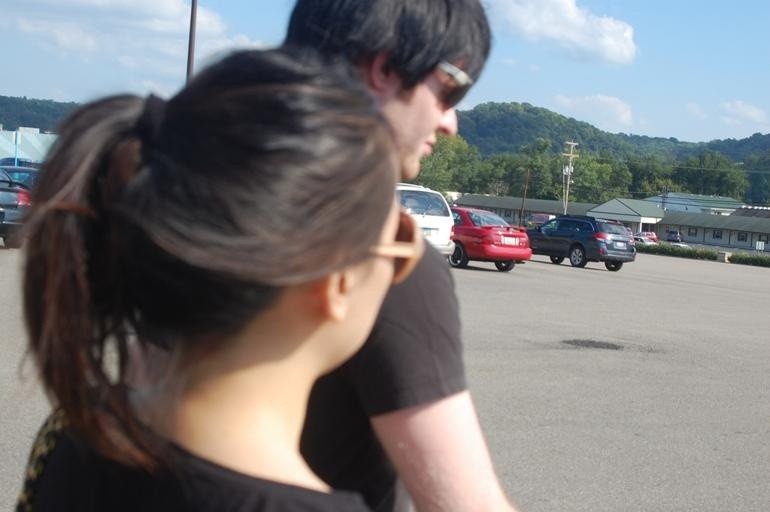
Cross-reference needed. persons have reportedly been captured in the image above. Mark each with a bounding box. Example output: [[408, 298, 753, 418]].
[[14, 46, 428, 512], [274, 3, 520, 511]]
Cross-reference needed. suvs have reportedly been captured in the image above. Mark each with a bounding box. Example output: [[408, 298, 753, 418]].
[[527, 215, 635, 271], [395, 182, 455, 263]]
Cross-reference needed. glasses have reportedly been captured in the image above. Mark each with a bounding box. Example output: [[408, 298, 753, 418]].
[[367, 212, 422, 284], [437, 58, 474, 110]]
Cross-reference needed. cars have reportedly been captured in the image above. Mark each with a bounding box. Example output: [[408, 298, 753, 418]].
[[667, 231, 684, 242], [449, 206, 532, 271], [0, 165, 40, 248], [626, 227, 659, 246]]
